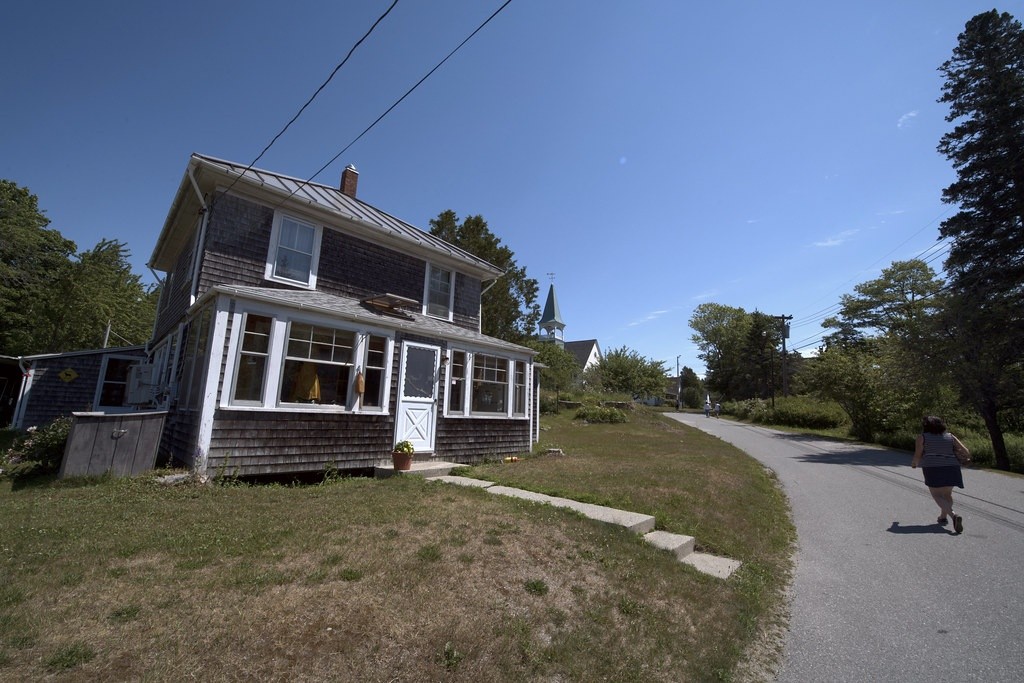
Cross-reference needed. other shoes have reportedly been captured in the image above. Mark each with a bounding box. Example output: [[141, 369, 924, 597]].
[[953, 514, 963, 532], [938, 517, 948, 525]]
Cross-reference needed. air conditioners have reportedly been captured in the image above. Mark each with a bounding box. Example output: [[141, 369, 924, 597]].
[[124, 363, 161, 404]]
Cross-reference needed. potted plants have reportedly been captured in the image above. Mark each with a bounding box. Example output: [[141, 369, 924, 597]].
[[392, 441, 414, 470]]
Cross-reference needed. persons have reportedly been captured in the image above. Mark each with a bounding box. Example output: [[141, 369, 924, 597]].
[[715, 400, 721, 418], [911, 416, 970, 533], [704, 402, 709, 418]]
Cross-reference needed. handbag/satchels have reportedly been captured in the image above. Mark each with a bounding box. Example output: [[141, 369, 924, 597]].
[[951, 436, 970, 463]]
